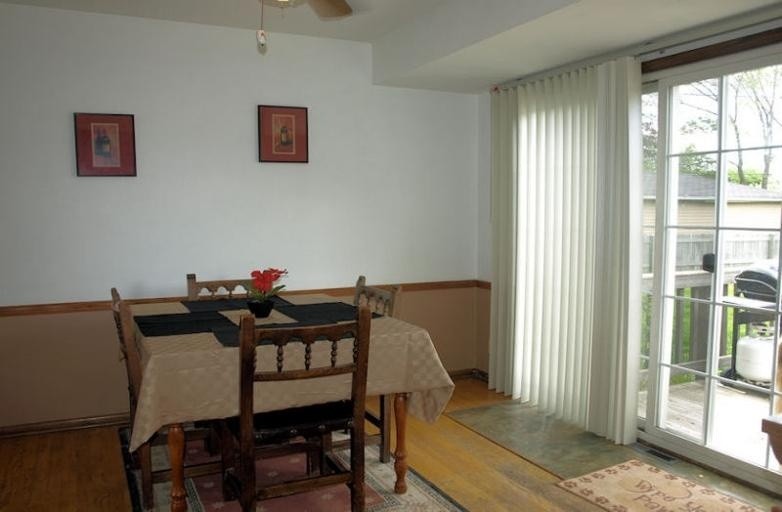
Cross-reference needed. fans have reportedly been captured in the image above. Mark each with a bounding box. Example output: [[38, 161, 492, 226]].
[[258, 0, 354, 20]]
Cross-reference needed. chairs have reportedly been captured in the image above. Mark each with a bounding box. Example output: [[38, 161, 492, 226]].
[[307, 276, 402, 476], [212, 306, 372, 512], [186, 272, 257, 455], [107, 287, 238, 510]]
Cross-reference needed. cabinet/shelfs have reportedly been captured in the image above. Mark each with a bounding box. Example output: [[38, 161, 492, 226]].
[[721, 267, 779, 398]]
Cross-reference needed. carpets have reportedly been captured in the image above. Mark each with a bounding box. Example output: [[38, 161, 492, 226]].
[[445, 398, 782, 512], [553, 458, 764, 512], [119, 423, 469, 512]]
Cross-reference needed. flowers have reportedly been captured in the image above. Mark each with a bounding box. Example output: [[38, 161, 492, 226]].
[[242, 268, 288, 301]]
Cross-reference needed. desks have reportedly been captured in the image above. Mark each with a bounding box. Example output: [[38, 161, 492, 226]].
[[107, 292, 455, 509]]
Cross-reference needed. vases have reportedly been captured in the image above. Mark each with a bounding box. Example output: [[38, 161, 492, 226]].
[[247, 300, 275, 317]]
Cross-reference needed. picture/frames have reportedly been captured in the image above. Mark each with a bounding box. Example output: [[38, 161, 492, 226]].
[[73, 112, 137, 177], [257, 104, 308, 164]]
